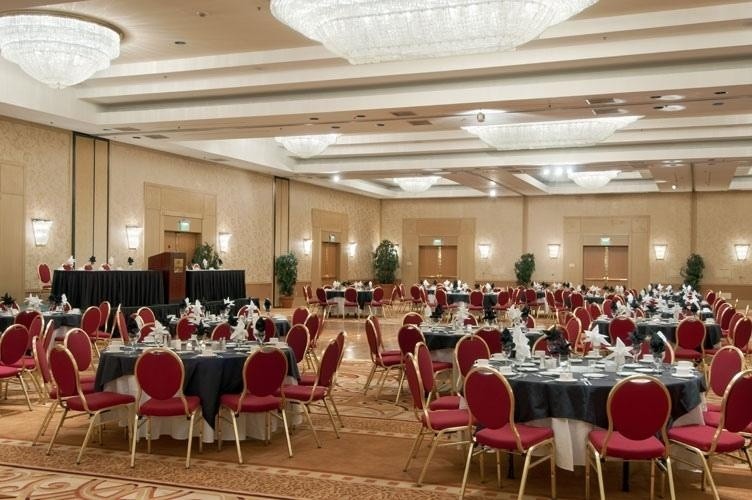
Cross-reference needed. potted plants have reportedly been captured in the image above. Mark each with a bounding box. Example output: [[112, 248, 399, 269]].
[[679, 253, 705, 291], [371, 239, 400, 300], [513, 253, 536, 289], [275, 253, 298, 308]]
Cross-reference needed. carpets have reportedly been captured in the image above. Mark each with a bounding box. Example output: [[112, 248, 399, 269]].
[[0, 358, 750, 500]]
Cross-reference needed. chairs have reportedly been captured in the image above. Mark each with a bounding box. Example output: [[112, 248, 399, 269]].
[[46, 344, 139, 466], [130, 344, 204, 466], [299, 331, 345, 428], [412, 340, 470, 450], [192, 263, 201, 270], [404, 350, 488, 487], [301, 282, 751, 404], [0, 298, 327, 412], [459, 365, 557, 499], [658, 367, 752, 498], [36, 263, 113, 308], [276, 338, 341, 448], [585, 374, 676, 500], [702, 346, 751, 464], [215, 343, 293, 464], [31, 327, 110, 447]]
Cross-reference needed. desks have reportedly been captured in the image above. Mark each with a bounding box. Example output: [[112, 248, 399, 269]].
[[92, 341, 290, 441], [48, 270, 165, 310], [186, 269, 246, 303], [476, 356, 706, 491]]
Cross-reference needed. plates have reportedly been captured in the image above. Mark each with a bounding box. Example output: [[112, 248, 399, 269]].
[[473, 355, 695, 383], [420, 324, 514, 334], [105, 340, 284, 358]]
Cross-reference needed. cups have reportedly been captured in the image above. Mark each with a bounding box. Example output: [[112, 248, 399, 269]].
[[108, 336, 288, 356], [474, 351, 694, 381]]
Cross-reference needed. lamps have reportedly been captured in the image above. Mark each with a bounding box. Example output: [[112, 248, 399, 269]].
[[126, 224, 142, 250], [348, 242, 357, 257], [31, 218, 52, 247], [734, 243, 750, 261], [275, 115, 645, 193], [303, 238, 313, 255], [478, 244, 491, 259], [653, 243, 668, 261], [219, 231, 232, 252], [547, 243, 561, 259], [269, 0, 598, 66], [0, 10, 124, 92]]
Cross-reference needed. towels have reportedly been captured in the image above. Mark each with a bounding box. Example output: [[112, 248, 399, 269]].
[[67, 255, 136, 266], [202, 258, 223, 269]]
